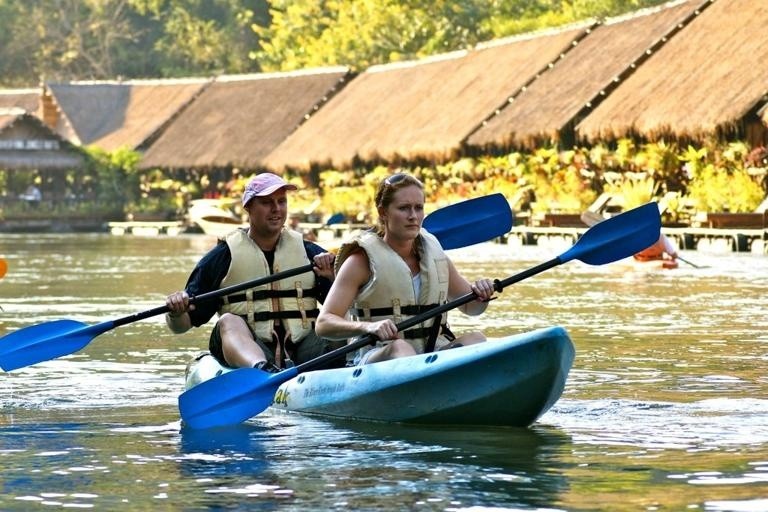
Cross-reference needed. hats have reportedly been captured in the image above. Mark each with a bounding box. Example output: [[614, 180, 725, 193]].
[[240, 172, 299, 208]]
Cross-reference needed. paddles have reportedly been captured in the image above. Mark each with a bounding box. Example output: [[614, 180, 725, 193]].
[[0, 193, 513, 371], [581, 210, 696, 268], [179, 202, 661, 429]]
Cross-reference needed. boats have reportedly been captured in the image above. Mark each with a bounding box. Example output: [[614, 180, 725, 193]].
[[606, 259, 679, 273], [181, 323, 576, 431], [190, 212, 249, 240]]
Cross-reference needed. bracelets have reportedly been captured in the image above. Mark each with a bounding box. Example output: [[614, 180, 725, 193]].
[[168, 311, 185, 318]]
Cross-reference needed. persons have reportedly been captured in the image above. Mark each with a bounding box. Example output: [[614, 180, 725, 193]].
[[315, 173, 494, 367], [25, 185, 41, 208], [165, 172, 347, 373], [632, 232, 678, 261]]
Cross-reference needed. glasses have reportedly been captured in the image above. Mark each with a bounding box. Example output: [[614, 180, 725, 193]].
[[379, 172, 424, 207]]
